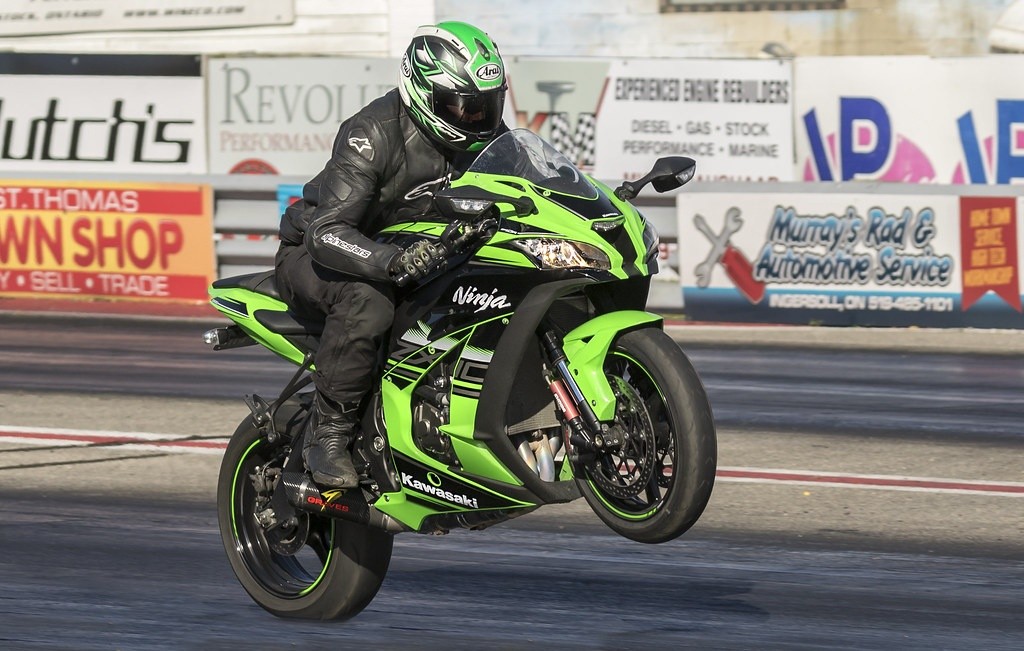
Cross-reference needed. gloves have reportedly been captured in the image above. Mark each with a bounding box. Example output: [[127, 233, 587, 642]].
[[392, 240, 442, 282]]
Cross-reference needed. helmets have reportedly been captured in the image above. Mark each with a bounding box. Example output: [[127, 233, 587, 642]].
[[396, 22, 507, 153]]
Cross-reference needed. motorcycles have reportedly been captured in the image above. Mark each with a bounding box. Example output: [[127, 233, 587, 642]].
[[199, 127, 721, 622]]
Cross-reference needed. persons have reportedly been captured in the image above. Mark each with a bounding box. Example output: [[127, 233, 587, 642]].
[[271, 19, 547, 492]]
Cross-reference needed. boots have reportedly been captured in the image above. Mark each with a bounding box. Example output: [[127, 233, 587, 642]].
[[301, 395, 359, 491]]
